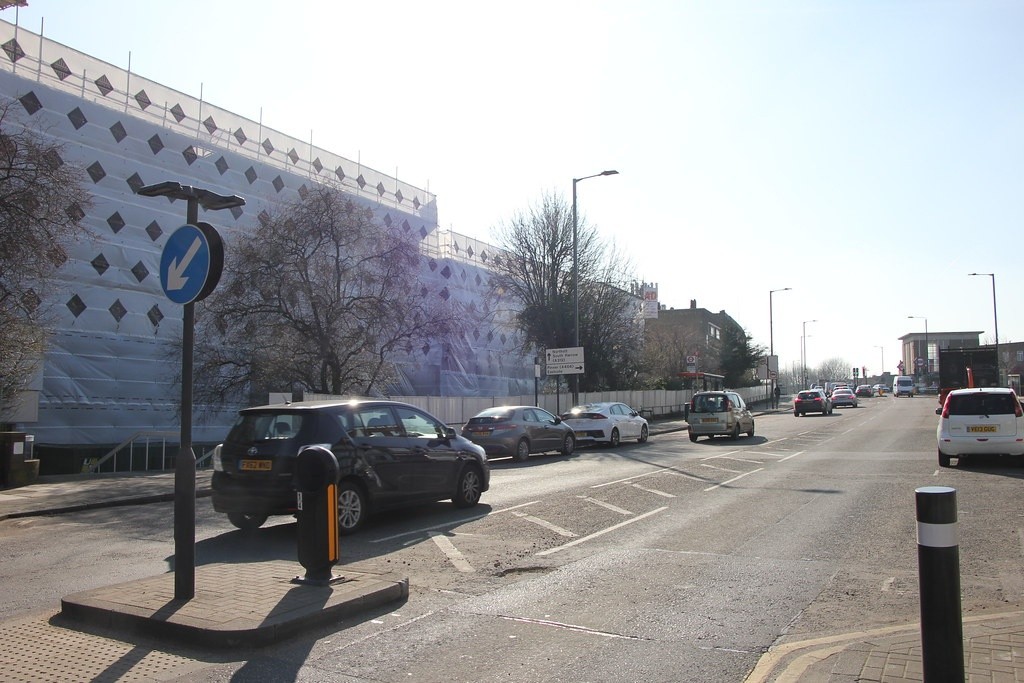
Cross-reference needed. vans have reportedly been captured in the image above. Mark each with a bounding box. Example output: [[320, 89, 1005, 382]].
[[892, 375, 914, 398], [687, 389, 756, 444], [209, 397, 491, 530]]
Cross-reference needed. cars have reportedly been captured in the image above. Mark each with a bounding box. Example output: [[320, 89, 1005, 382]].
[[794, 389, 833, 417], [461, 405, 576, 457], [936, 388, 1023, 468], [560, 401, 648, 447], [821, 379, 889, 407]]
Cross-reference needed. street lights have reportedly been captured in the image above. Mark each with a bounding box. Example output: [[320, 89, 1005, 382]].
[[769, 287, 792, 407], [801, 319, 816, 393], [572, 168, 619, 407], [968, 272, 1000, 387], [908, 315, 929, 374]]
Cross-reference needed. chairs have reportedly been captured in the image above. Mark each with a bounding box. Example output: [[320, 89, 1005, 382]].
[[271, 400, 727, 439]]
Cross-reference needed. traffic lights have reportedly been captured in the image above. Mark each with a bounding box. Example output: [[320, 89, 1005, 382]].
[[853, 368, 855, 376], [856, 368, 858, 376]]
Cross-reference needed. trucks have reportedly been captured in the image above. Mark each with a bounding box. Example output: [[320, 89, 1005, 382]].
[[934, 347, 1000, 416]]
